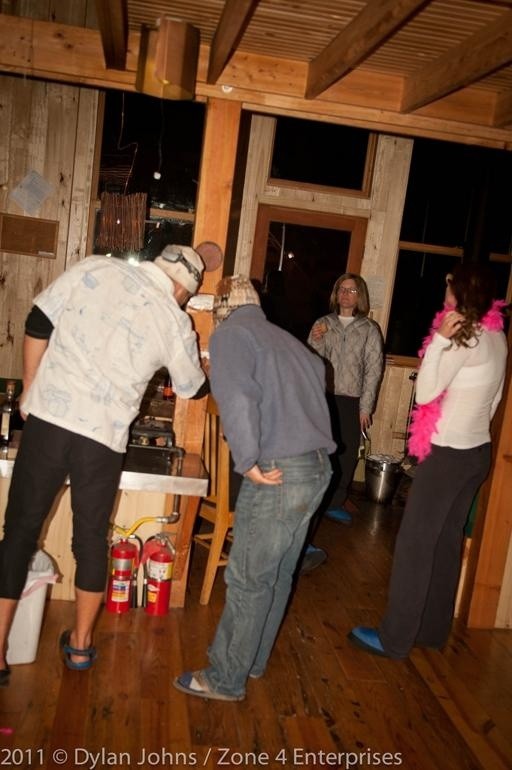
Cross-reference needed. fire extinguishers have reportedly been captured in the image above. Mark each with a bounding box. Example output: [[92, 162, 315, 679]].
[[105, 533, 176, 616]]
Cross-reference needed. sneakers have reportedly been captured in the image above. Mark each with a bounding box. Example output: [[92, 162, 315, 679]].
[[173, 669, 246, 701], [206, 642, 266, 678], [347, 626, 388, 655], [299, 544, 327, 571], [325, 509, 353, 521]]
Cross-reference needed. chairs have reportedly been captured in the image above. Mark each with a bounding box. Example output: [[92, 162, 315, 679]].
[[193, 393, 237, 609]]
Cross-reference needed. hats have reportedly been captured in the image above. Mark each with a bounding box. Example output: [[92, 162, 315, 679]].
[[211, 272, 262, 322], [155, 243, 205, 294]]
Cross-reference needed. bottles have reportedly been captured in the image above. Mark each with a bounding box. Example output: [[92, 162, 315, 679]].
[[0, 378, 18, 447]]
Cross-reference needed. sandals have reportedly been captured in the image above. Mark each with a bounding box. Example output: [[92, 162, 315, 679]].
[[59, 629, 96, 670], [0, 657, 12, 684]]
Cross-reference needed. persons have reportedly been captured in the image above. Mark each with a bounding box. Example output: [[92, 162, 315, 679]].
[[173, 274, 337, 702], [0, 244, 210, 679], [347, 259, 509, 661], [307, 274, 384, 523]]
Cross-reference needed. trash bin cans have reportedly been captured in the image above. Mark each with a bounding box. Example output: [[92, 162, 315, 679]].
[[365, 459, 401, 504], [3, 549, 55, 666]]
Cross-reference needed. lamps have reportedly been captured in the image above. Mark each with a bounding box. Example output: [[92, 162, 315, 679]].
[[133, 15, 202, 104]]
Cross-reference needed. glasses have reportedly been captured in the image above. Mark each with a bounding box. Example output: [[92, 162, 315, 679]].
[[339, 287, 359, 295]]
